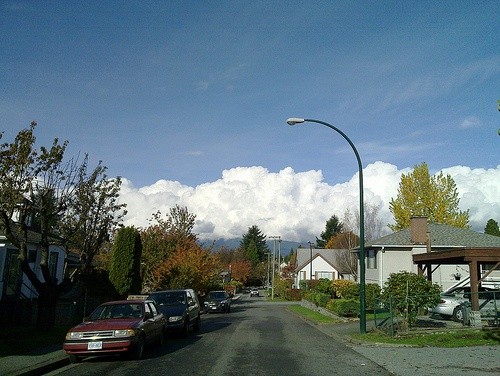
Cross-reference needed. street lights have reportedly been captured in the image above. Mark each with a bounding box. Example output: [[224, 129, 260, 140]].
[[287, 118, 366, 332]]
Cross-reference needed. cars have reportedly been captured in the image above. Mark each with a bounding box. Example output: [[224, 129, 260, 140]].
[[147, 288, 201, 336], [205, 291, 231, 314], [430, 287, 500, 320], [64, 296, 166, 362], [250, 288, 258, 295]]
